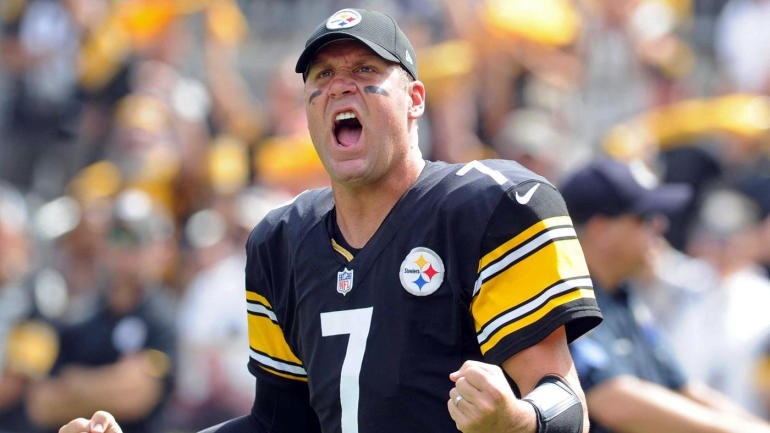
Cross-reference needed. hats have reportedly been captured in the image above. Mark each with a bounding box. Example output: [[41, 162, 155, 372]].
[[295, 8, 417, 81], [559, 157, 692, 220]]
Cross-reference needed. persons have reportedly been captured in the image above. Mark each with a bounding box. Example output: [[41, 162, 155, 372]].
[[0, 0, 403, 433], [58, 8, 603, 433], [402, 0, 770, 433]]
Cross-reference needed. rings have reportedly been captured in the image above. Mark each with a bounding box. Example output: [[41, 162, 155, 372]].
[[455, 396, 463, 408]]
